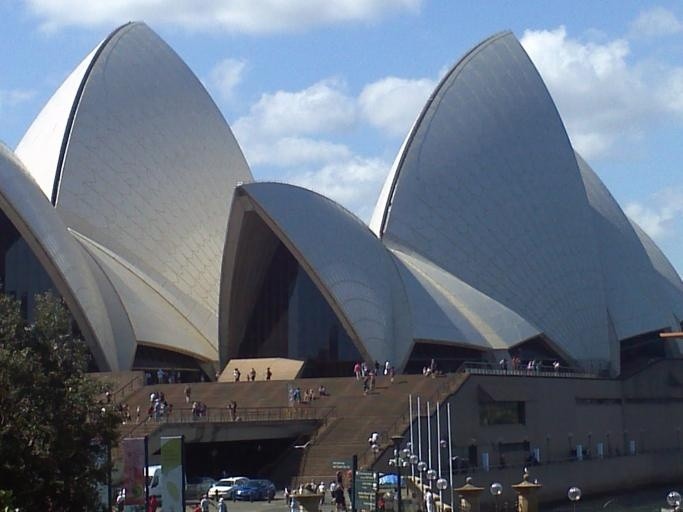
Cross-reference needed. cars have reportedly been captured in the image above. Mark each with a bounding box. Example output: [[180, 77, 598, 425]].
[[233, 480, 275, 502], [208, 476, 249, 499]]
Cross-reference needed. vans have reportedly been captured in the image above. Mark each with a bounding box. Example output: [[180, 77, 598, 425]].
[[117, 465, 186, 504]]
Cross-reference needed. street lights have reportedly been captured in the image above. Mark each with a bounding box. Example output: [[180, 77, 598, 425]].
[[389, 433, 406, 512]]
